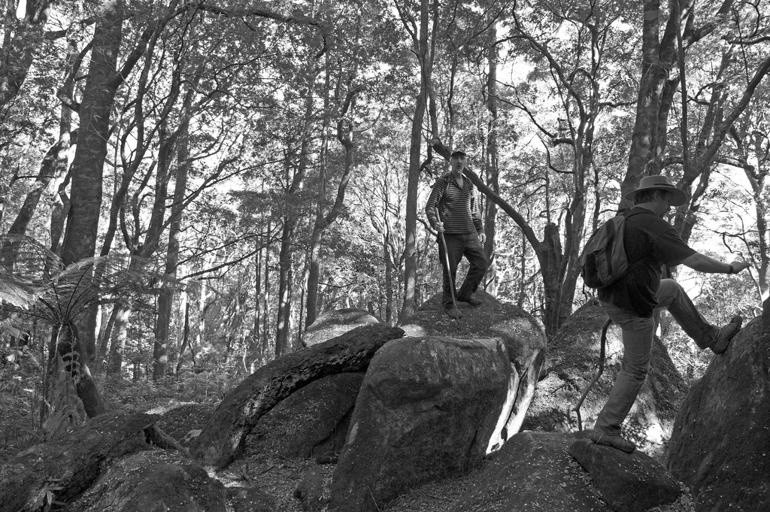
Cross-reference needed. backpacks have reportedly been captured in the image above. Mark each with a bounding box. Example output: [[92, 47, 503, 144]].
[[578, 208, 655, 290]]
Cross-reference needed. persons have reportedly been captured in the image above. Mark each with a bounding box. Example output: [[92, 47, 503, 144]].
[[587, 174, 753, 455], [424, 148, 490, 311]]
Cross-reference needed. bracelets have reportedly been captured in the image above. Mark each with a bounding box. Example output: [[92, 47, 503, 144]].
[[727, 263, 734, 274]]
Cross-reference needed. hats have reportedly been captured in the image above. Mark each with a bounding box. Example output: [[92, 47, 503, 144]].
[[623, 175, 687, 207], [452, 148, 466, 156]]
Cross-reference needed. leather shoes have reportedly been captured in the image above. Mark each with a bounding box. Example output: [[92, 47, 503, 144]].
[[711, 316, 744, 355], [456, 290, 483, 306], [591, 424, 636, 454], [444, 306, 463, 318]]
